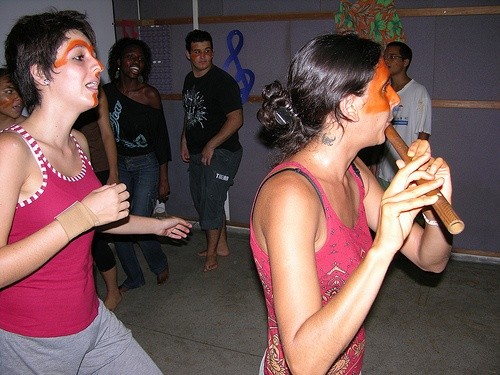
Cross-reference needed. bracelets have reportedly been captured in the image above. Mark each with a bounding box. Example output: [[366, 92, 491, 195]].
[[422, 212, 440, 227]]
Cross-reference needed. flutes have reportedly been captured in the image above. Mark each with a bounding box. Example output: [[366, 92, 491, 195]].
[[384, 123, 466, 236]]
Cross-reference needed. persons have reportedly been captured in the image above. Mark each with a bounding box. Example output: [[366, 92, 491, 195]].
[[181, 30, 244, 272], [250, 33, 453, 375], [0, 11, 193, 375], [370, 41, 432, 229], [71, 85, 122, 312], [103, 38, 171, 293], [0, 68, 28, 132]]
